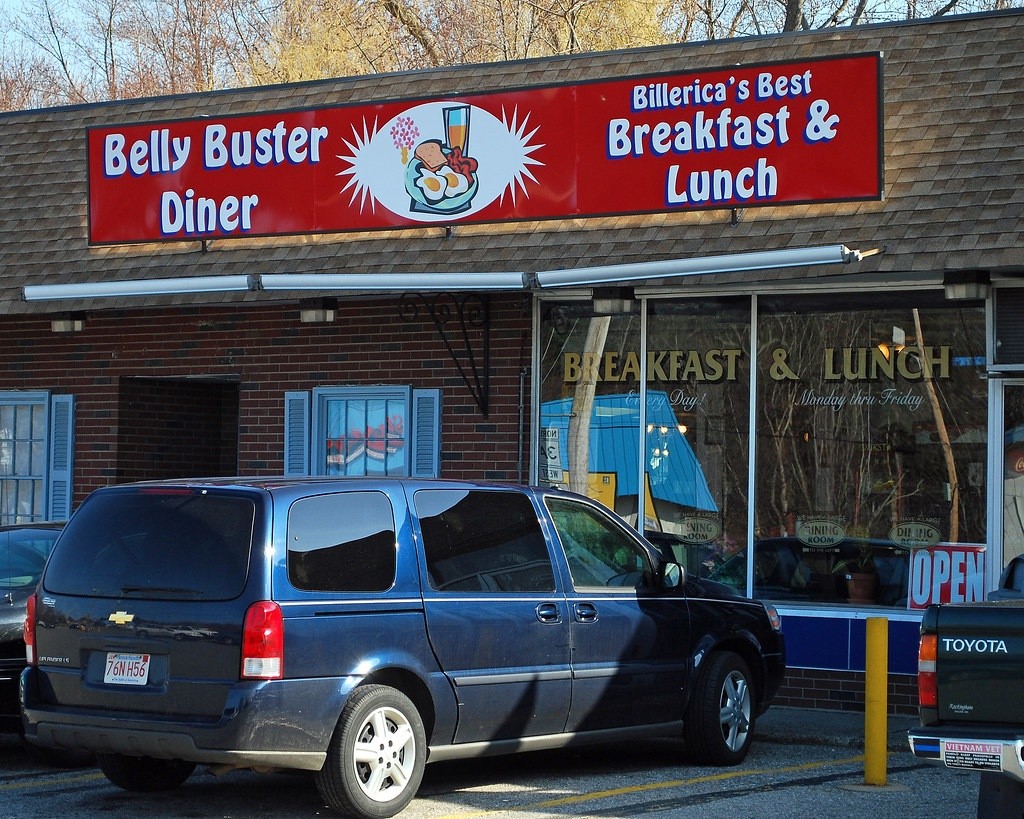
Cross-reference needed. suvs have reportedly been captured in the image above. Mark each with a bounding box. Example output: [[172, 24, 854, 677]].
[[705, 537, 910, 610], [19, 473, 788, 819]]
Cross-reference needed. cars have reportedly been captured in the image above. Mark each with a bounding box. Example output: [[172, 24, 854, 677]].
[[0, 528, 64, 726]]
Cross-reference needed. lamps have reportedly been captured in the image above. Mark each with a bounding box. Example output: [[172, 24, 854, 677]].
[[534, 244, 855, 288], [256, 271, 532, 290], [20, 275, 259, 303], [589, 286, 635, 313], [941, 269, 992, 301], [297, 296, 337, 323], [50, 310, 86, 333]]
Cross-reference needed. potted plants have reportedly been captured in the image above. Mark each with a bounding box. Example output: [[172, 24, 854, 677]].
[[830, 524, 882, 604]]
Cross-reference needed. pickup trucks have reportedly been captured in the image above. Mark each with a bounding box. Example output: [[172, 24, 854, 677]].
[[906, 553, 1024, 819]]
